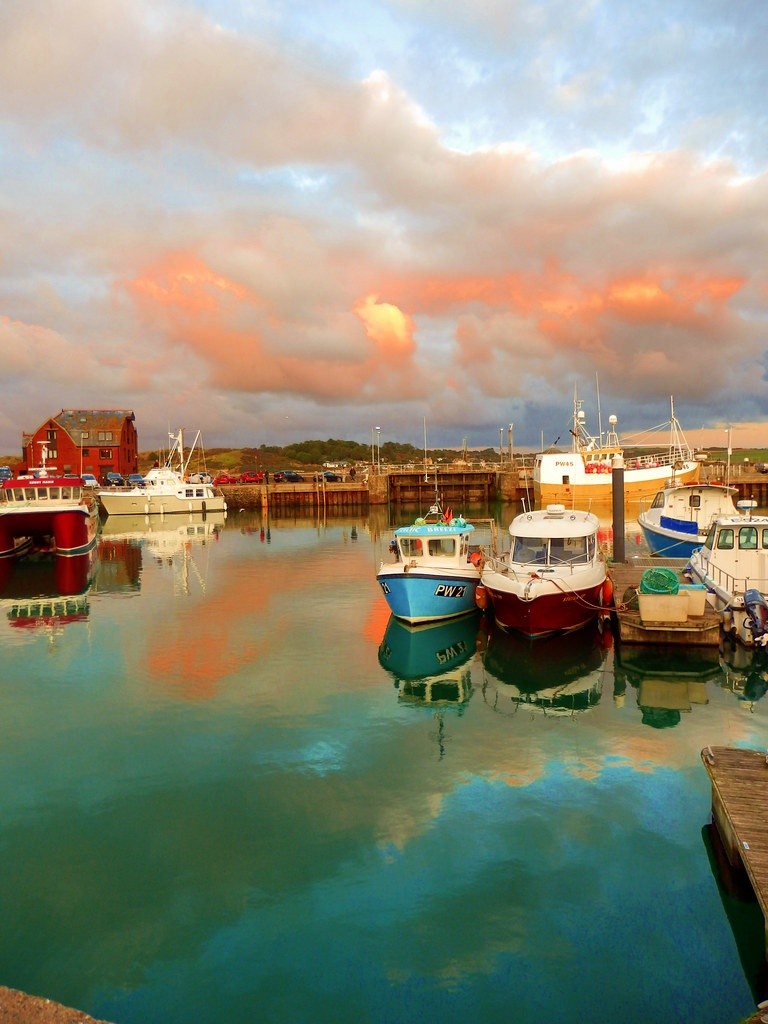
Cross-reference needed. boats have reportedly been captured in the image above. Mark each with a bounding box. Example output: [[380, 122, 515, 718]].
[[481, 453, 610, 636], [95, 427, 227, 514], [0, 432, 102, 554], [101, 511, 228, 595], [717, 634, 768, 714], [0, 539, 101, 656], [689, 495, 767, 650], [482, 619, 610, 720], [534, 371, 702, 501], [378, 612, 485, 761], [376, 416, 498, 626], [635, 427, 767, 561]]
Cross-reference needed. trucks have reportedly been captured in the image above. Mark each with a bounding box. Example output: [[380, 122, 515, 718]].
[[0, 466, 13, 486]]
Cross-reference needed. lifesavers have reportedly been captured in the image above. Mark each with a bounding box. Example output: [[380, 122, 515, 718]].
[[710, 481, 727, 486], [183, 477, 187, 481], [683, 482, 703, 485]]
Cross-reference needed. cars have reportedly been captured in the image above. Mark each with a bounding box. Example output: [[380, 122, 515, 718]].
[[189, 472, 237, 483], [273, 471, 305, 483], [237, 471, 260, 483], [82, 474, 99, 486], [104, 471, 124, 486], [312, 472, 343, 483], [125, 474, 146, 487]]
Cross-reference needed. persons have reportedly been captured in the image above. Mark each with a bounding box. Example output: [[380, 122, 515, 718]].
[[350, 467, 356, 481], [265, 470, 270, 484], [341, 467, 346, 483]]
